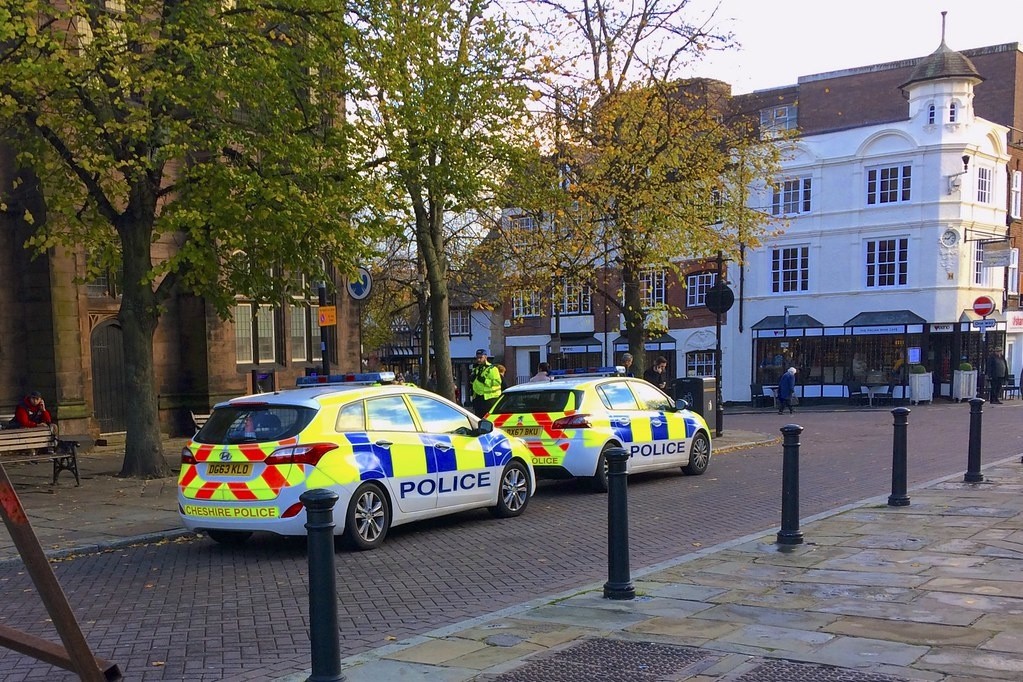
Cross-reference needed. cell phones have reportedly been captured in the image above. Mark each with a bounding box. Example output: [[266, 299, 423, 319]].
[[654, 364, 660, 370]]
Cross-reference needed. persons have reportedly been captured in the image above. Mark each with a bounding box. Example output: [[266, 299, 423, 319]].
[[985, 347, 1009, 404], [777, 367, 797, 415], [497, 365, 509, 391], [643, 355, 668, 391], [620, 353, 634, 377], [5, 391, 55, 455], [470, 349, 501, 418], [530, 362, 555, 402], [396, 371, 410, 383]]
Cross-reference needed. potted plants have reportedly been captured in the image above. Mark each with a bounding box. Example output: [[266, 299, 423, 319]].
[[953, 363, 976, 403], [910, 365, 933, 406]]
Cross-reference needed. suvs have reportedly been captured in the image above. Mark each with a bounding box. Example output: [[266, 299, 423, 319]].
[[175, 370, 538, 549], [485, 365, 712, 492]]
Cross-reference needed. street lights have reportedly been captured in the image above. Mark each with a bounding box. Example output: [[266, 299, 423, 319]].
[[782, 305, 799, 374]]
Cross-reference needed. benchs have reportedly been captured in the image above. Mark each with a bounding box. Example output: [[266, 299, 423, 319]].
[[0, 414, 56, 463], [188, 410, 250, 436], [0, 421, 81, 485], [977, 374, 1020, 400]]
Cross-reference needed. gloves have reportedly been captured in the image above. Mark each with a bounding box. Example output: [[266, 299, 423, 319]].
[[477, 376, 485, 383], [470, 375, 476, 381]]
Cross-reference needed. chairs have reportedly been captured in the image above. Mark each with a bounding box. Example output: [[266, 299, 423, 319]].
[[750, 383, 772, 408], [848, 380, 869, 405], [872, 381, 897, 407]]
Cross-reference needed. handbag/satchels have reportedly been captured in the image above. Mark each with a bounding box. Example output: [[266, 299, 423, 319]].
[[791, 392, 799, 405]]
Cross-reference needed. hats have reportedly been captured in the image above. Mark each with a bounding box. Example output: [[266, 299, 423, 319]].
[[993, 347, 1002, 351], [30, 391, 41, 398], [476, 349, 487, 355]]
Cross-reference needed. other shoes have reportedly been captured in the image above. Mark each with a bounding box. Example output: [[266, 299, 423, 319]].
[[28, 452, 35, 456], [778, 411, 783, 415], [790, 409, 796, 414], [47, 449, 54, 454], [990, 401, 1002, 404]]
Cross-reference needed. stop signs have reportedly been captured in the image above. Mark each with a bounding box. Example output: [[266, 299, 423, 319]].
[[973, 296, 994, 317]]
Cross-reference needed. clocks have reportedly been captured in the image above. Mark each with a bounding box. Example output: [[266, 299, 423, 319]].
[[942, 231, 956, 247]]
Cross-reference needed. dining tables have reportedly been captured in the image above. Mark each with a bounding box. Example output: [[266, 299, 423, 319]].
[[860, 382, 887, 408]]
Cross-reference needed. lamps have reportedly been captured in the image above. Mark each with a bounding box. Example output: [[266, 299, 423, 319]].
[[961, 154, 971, 173]]
